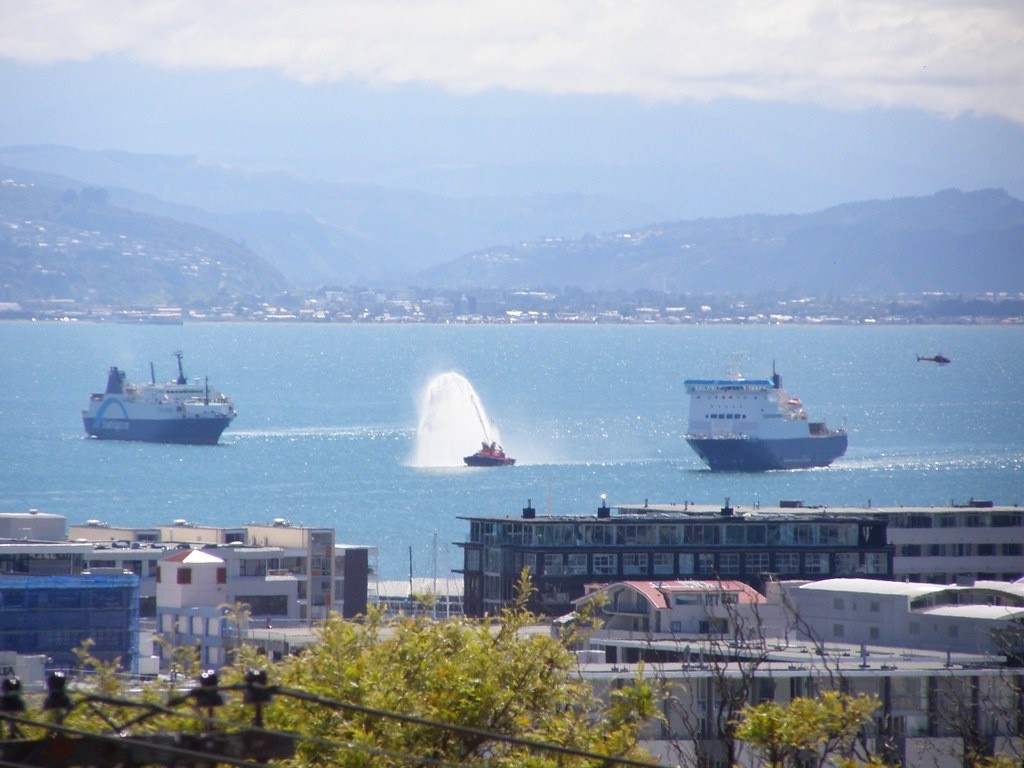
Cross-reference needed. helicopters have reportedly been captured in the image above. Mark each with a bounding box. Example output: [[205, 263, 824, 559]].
[[915, 350, 950, 364]]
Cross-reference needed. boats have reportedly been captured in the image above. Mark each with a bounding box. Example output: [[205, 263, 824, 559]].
[[462, 441, 516, 468], [82, 348, 236, 445], [681, 358, 849, 472]]
